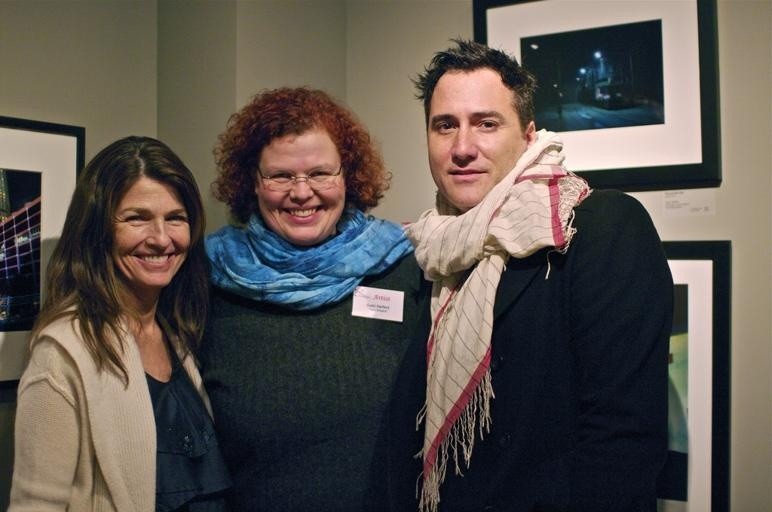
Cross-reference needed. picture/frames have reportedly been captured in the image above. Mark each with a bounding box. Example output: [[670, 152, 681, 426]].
[[469, 0, 726, 193], [655, 238, 734, 512], [0, 115, 88, 389]]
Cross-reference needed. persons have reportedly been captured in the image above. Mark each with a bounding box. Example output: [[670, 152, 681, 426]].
[[197, 87, 431, 511], [403, 37, 675, 511], [8, 135, 234, 511]]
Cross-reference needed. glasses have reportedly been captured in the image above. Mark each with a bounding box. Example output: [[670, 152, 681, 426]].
[[258, 162, 342, 190]]
